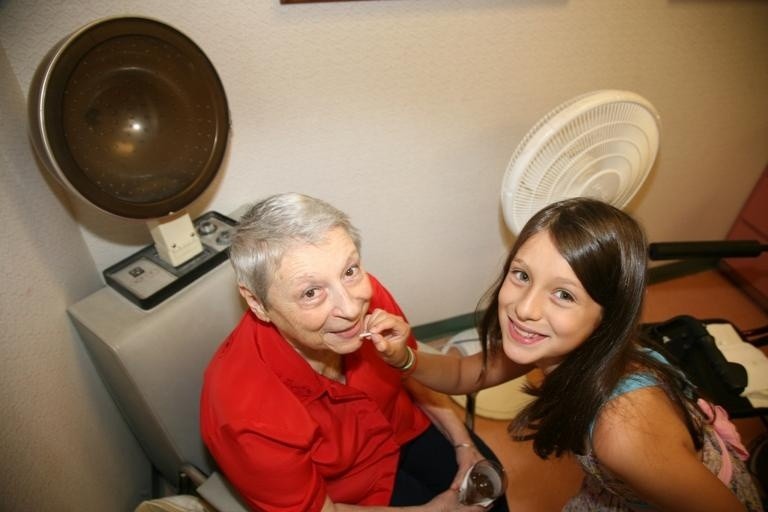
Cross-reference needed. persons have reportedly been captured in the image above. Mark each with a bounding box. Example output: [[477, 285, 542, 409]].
[[361, 196, 766, 511], [197, 186, 511, 512]]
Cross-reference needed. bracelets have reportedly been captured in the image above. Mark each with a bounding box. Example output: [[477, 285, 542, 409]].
[[452, 439, 476, 454], [397, 345, 419, 383]]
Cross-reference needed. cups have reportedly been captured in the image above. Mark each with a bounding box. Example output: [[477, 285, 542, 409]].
[[463, 459, 508, 506]]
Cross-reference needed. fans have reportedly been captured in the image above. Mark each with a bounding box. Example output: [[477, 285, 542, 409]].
[[439, 85, 664, 422]]
